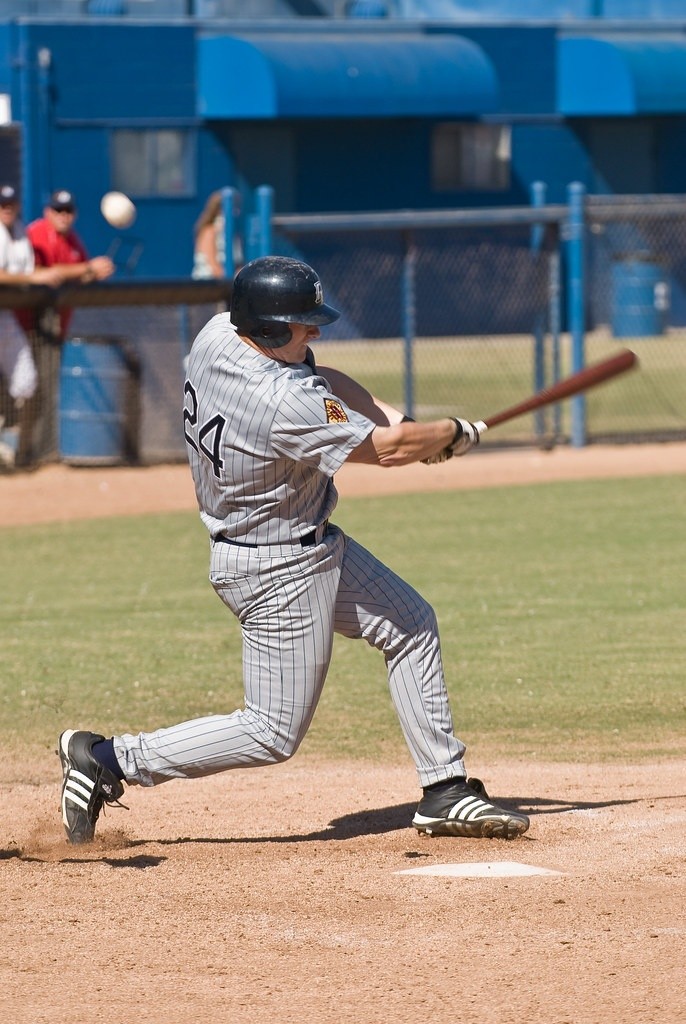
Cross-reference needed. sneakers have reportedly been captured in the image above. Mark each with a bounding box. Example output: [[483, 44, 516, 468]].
[[59, 728, 130, 845], [412, 777, 530, 839]]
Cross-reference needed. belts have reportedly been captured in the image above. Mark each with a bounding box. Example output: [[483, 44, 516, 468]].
[[216, 519, 328, 549]]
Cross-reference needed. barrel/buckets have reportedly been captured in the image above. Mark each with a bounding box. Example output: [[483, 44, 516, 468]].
[[58, 333, 134, 466], [611, 251, 668, 337]]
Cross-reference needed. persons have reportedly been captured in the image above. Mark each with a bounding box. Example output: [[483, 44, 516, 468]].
[[193, 186, 244, 280], [59, 256, 530, 844], [0, 185, 114, 474]]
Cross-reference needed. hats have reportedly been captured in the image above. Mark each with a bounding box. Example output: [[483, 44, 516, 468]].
[[230, 256, 341, 348], [0, 184, 19, 203], [47, 191, 75, 208]]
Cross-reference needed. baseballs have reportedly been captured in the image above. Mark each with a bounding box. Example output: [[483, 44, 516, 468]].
[[99, 190, 137, 228]]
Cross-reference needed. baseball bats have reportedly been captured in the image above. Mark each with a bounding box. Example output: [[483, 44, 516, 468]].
[[450, 351, 638, 456]]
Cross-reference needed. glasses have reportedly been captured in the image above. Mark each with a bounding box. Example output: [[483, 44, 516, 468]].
[[51, 205, 74, 213]]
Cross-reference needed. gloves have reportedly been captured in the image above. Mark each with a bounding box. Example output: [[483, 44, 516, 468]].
[[445, 417, 480, 457], [401, 415, 453, 464]]
